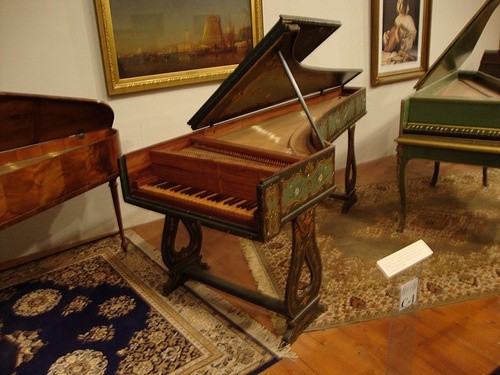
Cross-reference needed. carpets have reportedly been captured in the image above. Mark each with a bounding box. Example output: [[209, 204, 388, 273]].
[[0, 229, 298, 375], [237, 166, 500, 336]]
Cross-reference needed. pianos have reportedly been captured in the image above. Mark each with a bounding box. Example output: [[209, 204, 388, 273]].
[[0, 91, 128, 256], [116, 12, 367, 350], [393, 0, 500, 233]]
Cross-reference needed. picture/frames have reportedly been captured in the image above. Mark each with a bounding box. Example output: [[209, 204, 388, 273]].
[[369, 0, 433, 88], [91, 0, 264, 97]]
